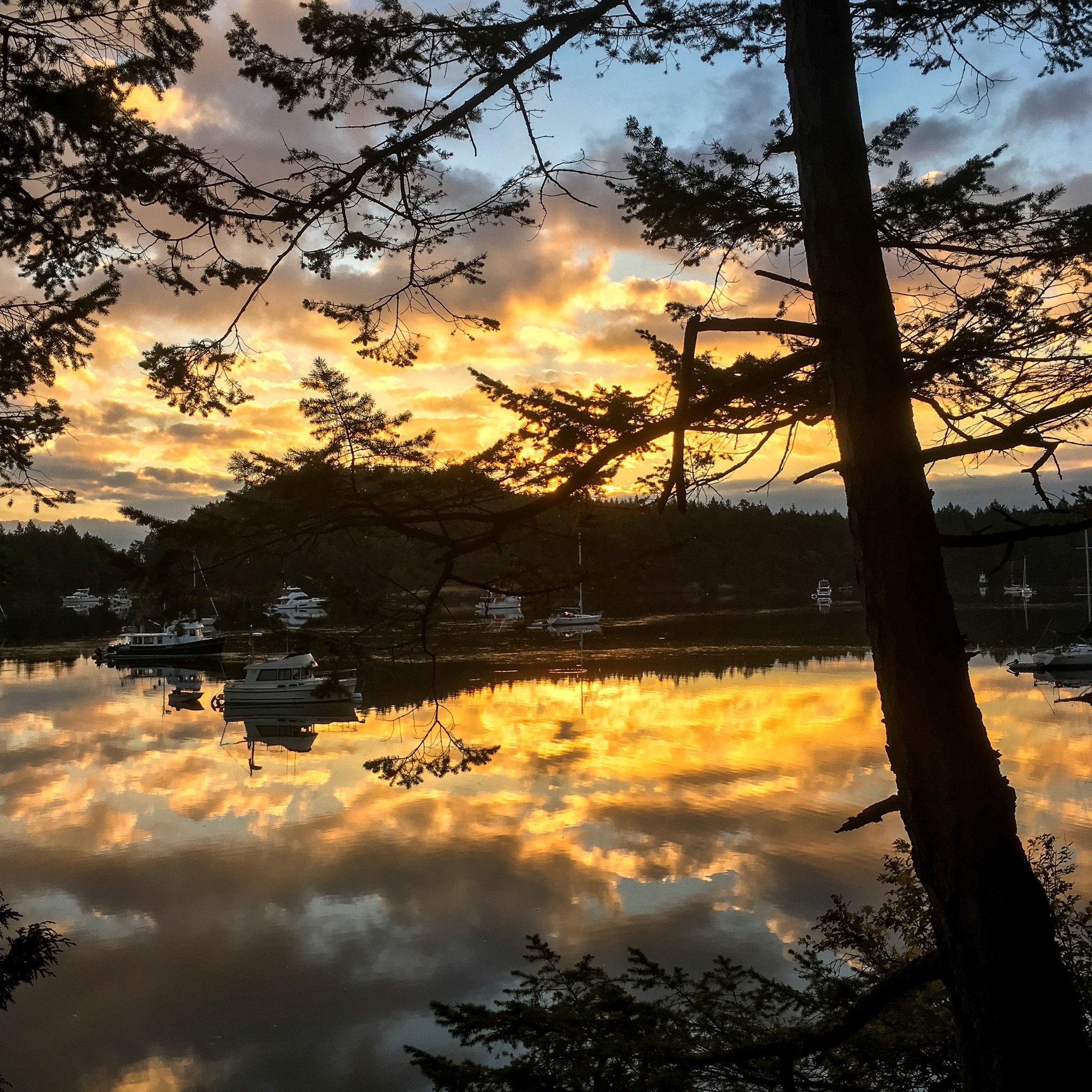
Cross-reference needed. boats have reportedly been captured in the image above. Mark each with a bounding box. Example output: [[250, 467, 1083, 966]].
[[547, 582, 602, 625], [108, 604, 132, 620], [108, 588, 132, 604], [168, 692, 204, 700], [475, 585, 524, 609], [1032, 642, 1092, 668], [268, 571, 330, 608], [61, 602, 104, 617], [60, 589, 104, 602], [168, 700, 205, 711], [223, 702, 359, 753], [475, 607, 524, 620], [92, 656, 227, 692], [211, 651, 359, 704], [1033, 662, 1092, 689], [92, 619, 229, 656], [263, 609, 330, 630], [816, 579, 832, 597], [547, 625, 603, 669], [817, 597, 831, 607]]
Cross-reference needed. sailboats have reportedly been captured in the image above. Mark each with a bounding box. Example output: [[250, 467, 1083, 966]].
[[1021, 557, 1033, 593], [1003, 561, 1022, 591], [1022, 593, 1032, 632]]
